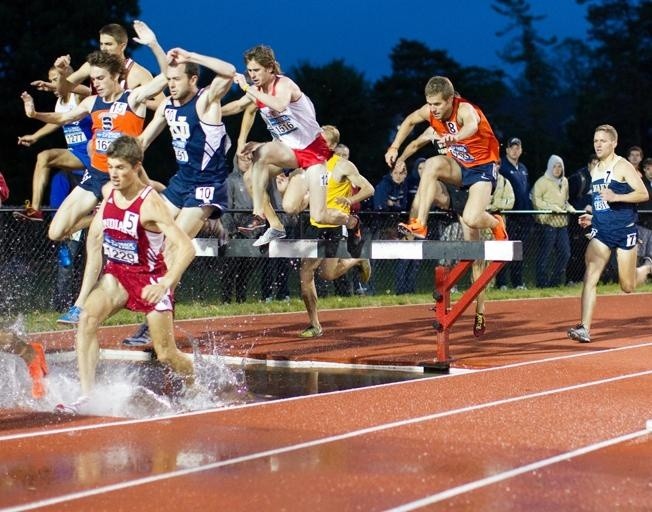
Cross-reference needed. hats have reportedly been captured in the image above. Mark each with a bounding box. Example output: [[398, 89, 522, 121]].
[[508, 137, 521, 146]]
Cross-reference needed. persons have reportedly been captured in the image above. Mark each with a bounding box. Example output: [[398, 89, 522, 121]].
[[564, 147, 652, 287], [1, 20, 514, 415], [529, 154, 575, 288], [495, 138, 533, 290], [568, 125, 652, 342]]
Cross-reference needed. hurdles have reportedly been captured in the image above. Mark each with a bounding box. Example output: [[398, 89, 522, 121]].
[[70, 227, 524, 361]]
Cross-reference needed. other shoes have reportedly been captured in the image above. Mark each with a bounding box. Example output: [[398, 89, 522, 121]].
[[27, 340, 47, 397]]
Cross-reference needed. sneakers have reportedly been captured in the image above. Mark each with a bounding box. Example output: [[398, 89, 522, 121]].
[[568, 325, 592, 343], [252, 227, 286, 247], [237, 214, 266, 234], [299, 325, 323, 338], [474, 312, 485, 337], [209, 217, 228, 241], [491, 215, 508, 241], [123, 323, 153, 346], [347, 215, 362, 249], [397, 221, 426, 239], [55, 307, 81, 325], [353, 259, 371, 282]]
